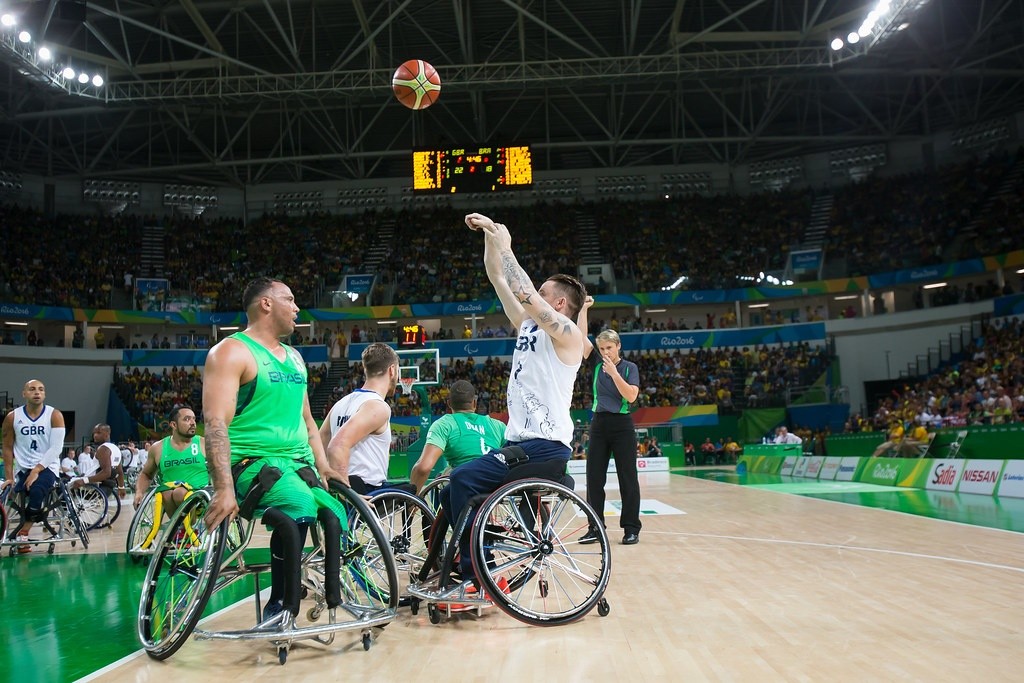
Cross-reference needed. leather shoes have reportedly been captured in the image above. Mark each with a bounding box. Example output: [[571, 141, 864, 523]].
[[622, 533, 639, 544], [578, 526, 599, 544]]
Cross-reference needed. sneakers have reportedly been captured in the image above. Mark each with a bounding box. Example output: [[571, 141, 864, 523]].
[[261, 600, 282, 643], [436, 577, 511, 611], [16, 533, 32, 553]]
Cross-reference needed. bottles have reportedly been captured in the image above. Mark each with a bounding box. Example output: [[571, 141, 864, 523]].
[[762, 437, 766, 444], [767, 437, 771, 444]]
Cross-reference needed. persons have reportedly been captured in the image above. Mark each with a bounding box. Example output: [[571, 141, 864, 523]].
[[576, 295, 642, 543], [899, 418, 928, 458], [0, 150, 1024, 481], [401, 380, 507, 568], [132, 407, 212, 549], [408, 213, 588, 610], [871, 419, 904, 457], [202, 276, 350, 625], [69, 424, 125, 500], [776, 427, 802, 444], [0, 379, 66, 552], [319, 343, 417, 513]]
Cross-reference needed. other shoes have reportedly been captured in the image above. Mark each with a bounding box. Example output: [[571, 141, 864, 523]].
[[170, 528, 198, 549]]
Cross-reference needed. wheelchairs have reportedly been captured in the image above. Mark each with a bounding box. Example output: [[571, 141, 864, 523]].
[[125, 462, 612, 665], [0, 474, 121, 557]]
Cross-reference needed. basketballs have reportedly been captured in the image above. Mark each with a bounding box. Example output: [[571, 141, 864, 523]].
[[393, 59, 441, 110]]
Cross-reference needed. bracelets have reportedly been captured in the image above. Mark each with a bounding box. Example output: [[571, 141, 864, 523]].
[[83, 477, 89, 484], [118, 486, 124, 489]]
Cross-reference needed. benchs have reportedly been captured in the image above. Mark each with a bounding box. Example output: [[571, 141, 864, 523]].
[[730, 365, 801, 409]]
